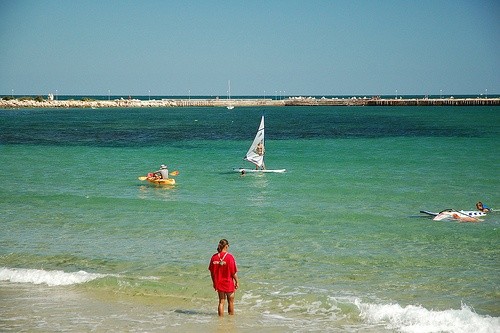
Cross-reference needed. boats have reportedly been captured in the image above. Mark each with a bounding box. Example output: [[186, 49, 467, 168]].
[[138, 177, 175, 184], [420, 209, 486, 222]]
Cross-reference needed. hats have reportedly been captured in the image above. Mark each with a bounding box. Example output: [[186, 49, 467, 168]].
[[160, 164, 167, 169]]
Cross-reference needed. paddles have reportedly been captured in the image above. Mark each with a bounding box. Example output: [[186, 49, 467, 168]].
[[138, 171, 179, 181]]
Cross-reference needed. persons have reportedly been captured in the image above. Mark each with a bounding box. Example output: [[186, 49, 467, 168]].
[[476, 200, 490, 212], [207, 239, 239, 317], [256, 142, 265, 169], [152, 164, 168, 180]]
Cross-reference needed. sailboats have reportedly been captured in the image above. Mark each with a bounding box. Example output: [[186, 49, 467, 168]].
[[232, 116, 286, 172]]
